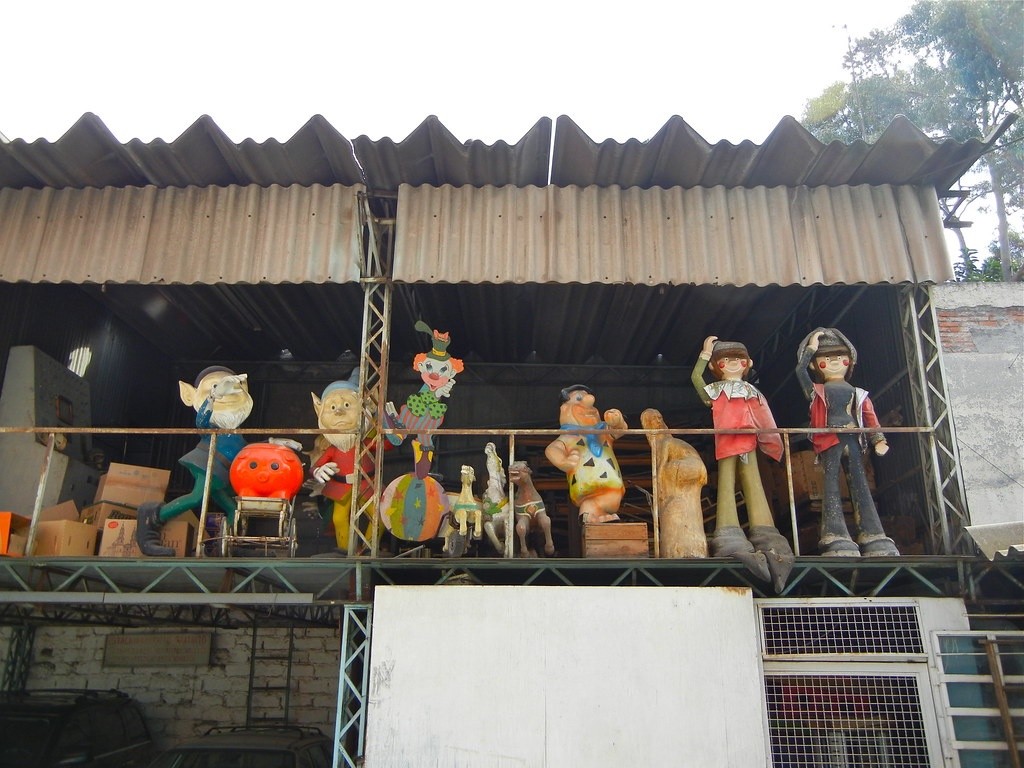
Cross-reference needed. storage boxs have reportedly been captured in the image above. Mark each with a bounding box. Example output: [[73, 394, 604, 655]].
[[779, 450, 876, 507], [570, 523, 650, 558], [0, 463, 226, 557]]
